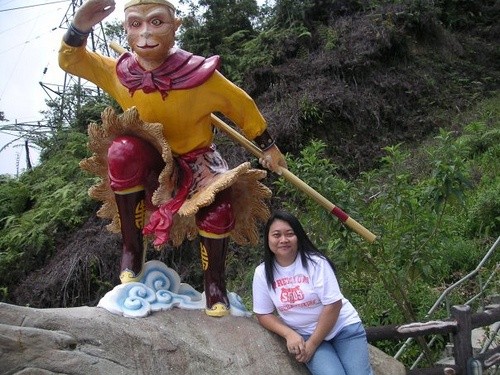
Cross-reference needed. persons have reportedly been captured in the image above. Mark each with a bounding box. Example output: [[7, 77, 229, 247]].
[[58, 0, 375, 317], [253, 212, 372, 375]]
